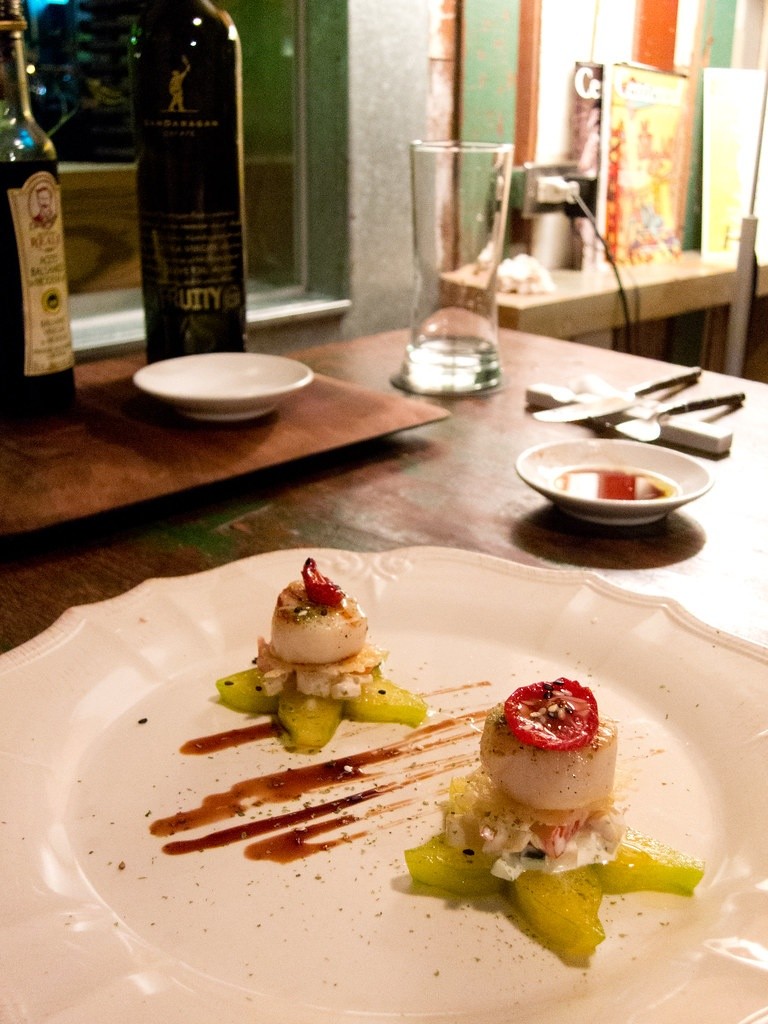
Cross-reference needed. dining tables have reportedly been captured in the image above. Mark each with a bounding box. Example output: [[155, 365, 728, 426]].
[[0, 321, 767, 1024]]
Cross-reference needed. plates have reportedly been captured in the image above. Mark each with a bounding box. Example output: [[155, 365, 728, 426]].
[[133, 353, 314, 420], [514, 438, 714, 526], [1, 545, 768, 1024]]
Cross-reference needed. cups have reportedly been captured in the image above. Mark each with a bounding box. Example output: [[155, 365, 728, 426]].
[[392, 141, 516, 397]]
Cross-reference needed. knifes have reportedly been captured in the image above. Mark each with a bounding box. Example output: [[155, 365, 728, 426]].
[[532, 366, 702, 423]]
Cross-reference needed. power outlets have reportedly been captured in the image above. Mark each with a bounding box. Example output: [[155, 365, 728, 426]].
[[521, 163, 577, 218]]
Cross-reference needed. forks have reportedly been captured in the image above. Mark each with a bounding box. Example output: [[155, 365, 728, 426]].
[[588, 391, 746, 442]]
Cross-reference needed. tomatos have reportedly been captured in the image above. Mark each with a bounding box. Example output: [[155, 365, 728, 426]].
[[505, 676, 600, 751], [302, 557, 346, 605]]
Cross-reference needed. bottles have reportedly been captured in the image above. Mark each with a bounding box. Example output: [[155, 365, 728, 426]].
[[0, 0, 76, 424], [132, 0, 245, 364]]
